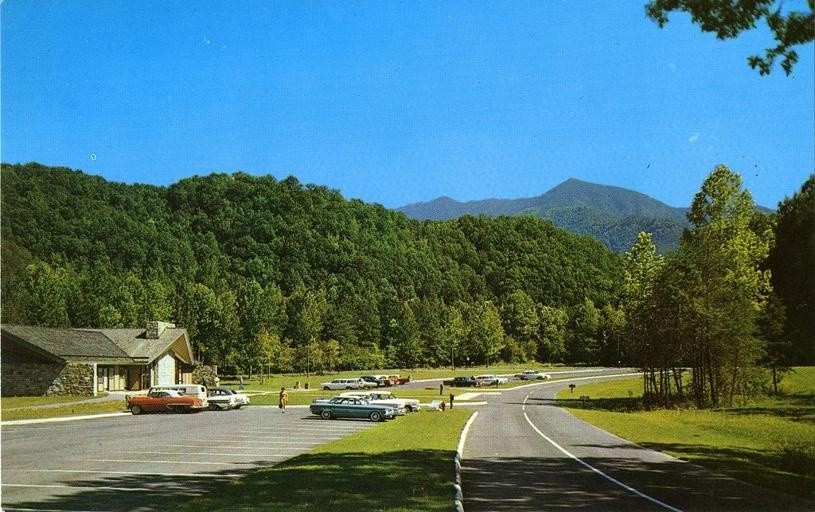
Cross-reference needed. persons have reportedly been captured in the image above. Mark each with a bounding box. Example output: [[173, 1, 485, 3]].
[[279, 388, 288, 413], [239, 376, 244, 390]]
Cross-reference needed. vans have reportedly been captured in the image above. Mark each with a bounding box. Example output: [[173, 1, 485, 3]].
[[148, 385, 210, 413]]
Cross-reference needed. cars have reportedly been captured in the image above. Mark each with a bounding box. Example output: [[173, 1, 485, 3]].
[[470, 375, 509, 386], [514, 371, 549, 380], [205, 386, 250, 411], [208, 391, 241, 410], [321, 372, 412, 390], [308, 399, 399, 422], [312, 395, 406, 419], [368, 391, 421, 414], [126, 390, 205, 416], [443, 376, 486, 388]]
